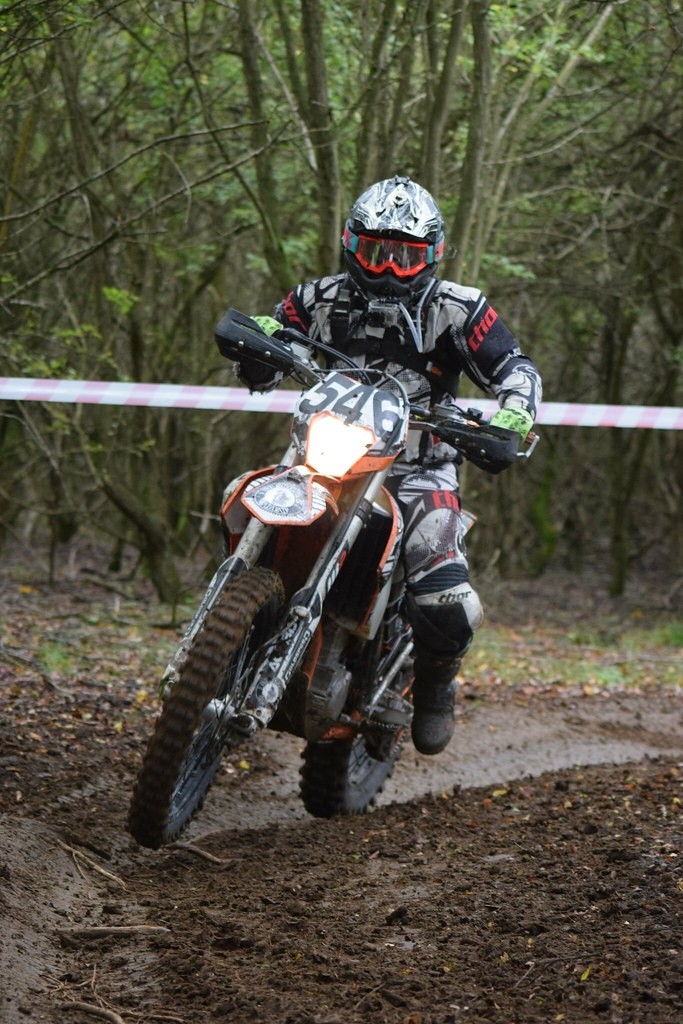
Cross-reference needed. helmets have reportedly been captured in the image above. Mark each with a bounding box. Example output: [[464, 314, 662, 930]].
[[342, 175, 444, 298]]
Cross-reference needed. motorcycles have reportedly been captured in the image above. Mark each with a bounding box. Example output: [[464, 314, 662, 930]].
[[127, 309, 539, 852]]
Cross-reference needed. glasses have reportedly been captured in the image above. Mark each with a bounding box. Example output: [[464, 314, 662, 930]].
[[356, 236, 435, 276]]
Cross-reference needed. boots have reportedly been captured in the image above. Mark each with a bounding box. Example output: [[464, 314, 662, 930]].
[[411, 602, 474, 755]]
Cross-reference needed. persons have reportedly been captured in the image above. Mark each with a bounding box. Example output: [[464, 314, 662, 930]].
[[231, 176, 544, 754]]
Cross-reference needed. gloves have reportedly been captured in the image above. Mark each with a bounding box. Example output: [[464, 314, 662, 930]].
[[486, 405, 534, 449], [239, 315, 284, 338]]
